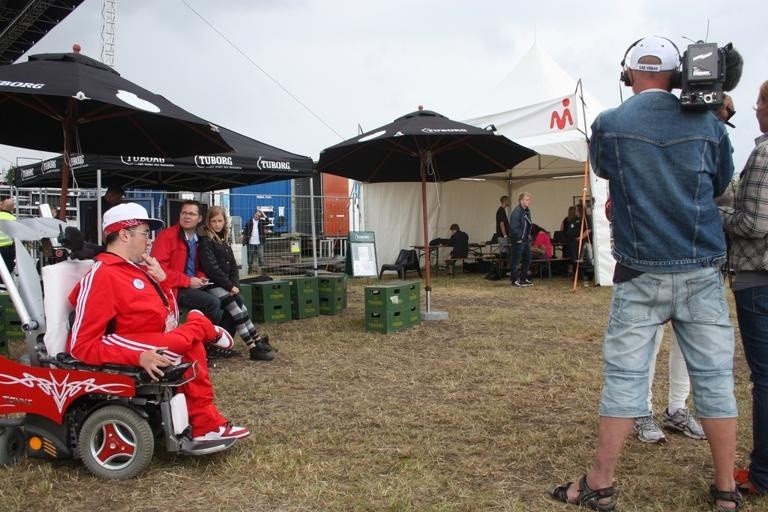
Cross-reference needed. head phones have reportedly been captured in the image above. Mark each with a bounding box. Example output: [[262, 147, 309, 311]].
[[621, 36, 680, 88]]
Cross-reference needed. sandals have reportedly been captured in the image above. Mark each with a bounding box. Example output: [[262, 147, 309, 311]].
[[549, 473, 617, 512], [709, 482, 745, 512], [733, 468, 765, 496]]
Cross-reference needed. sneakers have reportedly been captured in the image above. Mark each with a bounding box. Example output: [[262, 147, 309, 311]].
[[511, 279, 522, 288], [249, 334, 279, 361], [191, 421, 252, 441], [187, 308, 235, 351], [662, 406, 709, 440], [633, 411, 666, 443], [519, 278, 534, 287]]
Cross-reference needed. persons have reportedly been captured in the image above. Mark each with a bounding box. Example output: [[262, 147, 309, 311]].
[[198, 206, 279, 361], [497, 196, 511, 276], [84, 185, 126, 244], [66, 202, 249, 443], [508, 192, 533, 288], [148, 200, 244, 360], [714, 81, 768, 497], [526, 224, 552, 275], [548, 37, 745, 512], [48, 204, 67, 264], [633, 182, 735, 443], [242, 209, 270, 277], [560, 207, 576, 277], [0, 199, 16, 295], [438, 224, 469, 274], [567, 204, 592, 288]]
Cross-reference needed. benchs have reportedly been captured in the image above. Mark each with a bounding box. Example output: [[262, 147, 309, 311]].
[[408, 238, 574, 280]]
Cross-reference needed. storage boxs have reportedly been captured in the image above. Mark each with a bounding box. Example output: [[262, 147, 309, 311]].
[[238, 269, 348, 326], [362, 278, 422, 335]]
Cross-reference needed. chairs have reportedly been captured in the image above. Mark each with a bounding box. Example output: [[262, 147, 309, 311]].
[[379, 248, 422, 280]]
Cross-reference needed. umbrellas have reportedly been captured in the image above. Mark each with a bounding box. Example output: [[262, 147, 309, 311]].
[[0, 52, 238, 224], [317, 110, 540, 287]]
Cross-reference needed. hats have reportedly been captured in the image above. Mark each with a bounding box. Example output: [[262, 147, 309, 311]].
[[100, 201, 167, 232], [623, 35, 684, 75]]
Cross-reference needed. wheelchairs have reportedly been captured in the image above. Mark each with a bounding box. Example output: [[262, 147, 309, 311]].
[[0, 218, 239, 481]]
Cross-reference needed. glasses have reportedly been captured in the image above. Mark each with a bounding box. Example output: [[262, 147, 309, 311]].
[[178, 211, 200, 218]]
